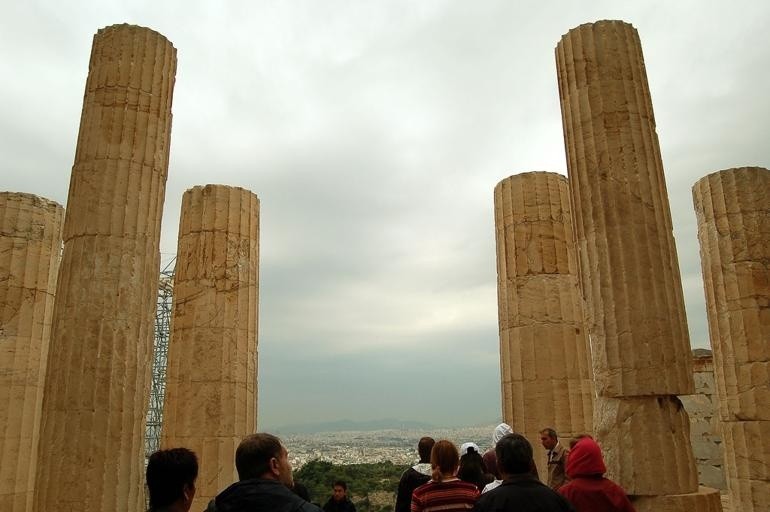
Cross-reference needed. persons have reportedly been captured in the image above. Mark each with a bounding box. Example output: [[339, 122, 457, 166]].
[[394, 422, 636, 511], [291, 482, 311, 503], [322, 480, 357, 512], [143, 447, 200, 512], [204, 433, 324, 511]]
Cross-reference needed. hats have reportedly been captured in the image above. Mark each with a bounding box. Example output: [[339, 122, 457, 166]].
[[459, 442, 480, 455]]
